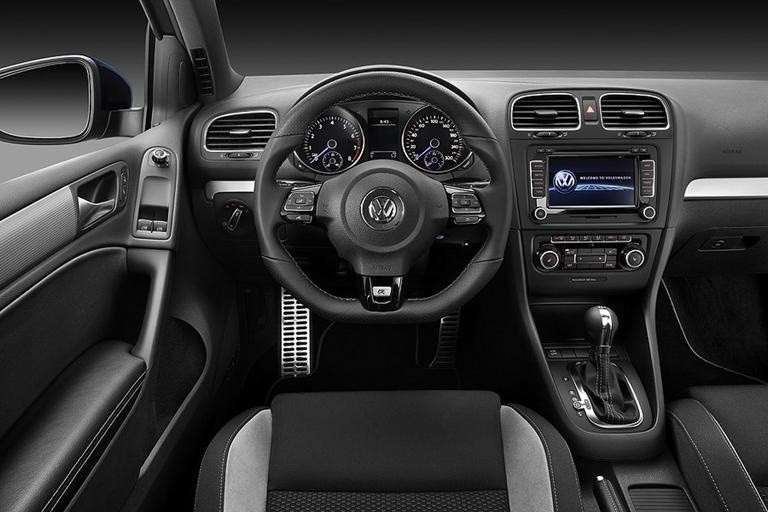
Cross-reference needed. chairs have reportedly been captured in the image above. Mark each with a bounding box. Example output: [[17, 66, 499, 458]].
[[184, 386, 590, 512]]
